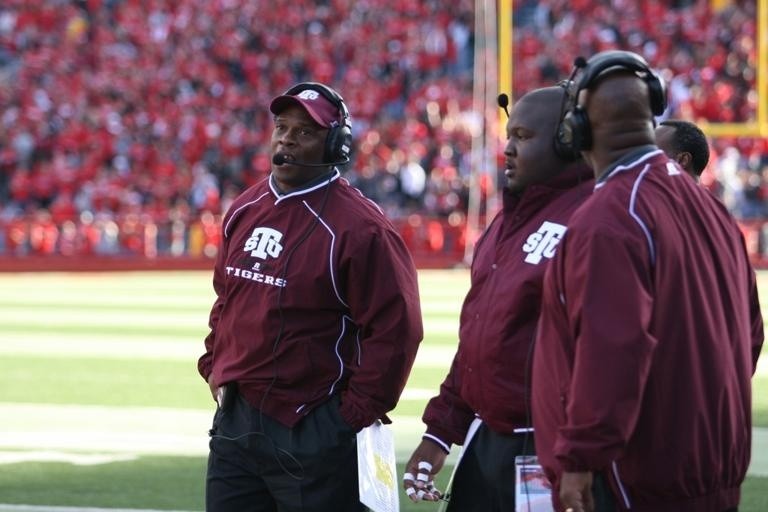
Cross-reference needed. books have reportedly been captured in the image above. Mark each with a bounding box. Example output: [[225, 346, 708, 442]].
[[515, 449, 575, 512]]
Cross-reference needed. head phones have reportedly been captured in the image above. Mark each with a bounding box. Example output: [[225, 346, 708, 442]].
[[557, 51, 667, 154], [281, 80, 354, 164]]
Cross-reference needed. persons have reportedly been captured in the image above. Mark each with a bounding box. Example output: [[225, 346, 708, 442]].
[[527, 49, 765, 512], [195, 82, 427, 512], [400, 82, 595, 512]]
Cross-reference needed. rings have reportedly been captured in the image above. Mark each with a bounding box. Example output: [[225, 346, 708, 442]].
[[403, 461, 438, 500], [563, 507, 574, 512]]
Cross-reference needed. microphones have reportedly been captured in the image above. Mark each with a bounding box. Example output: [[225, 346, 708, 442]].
[[272, 152, 352, 179], [553, 54, 587, 123], [495, 90, 514, 117]]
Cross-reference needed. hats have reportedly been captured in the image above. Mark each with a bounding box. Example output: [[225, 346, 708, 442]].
[[269, 83, 350, 129]]
